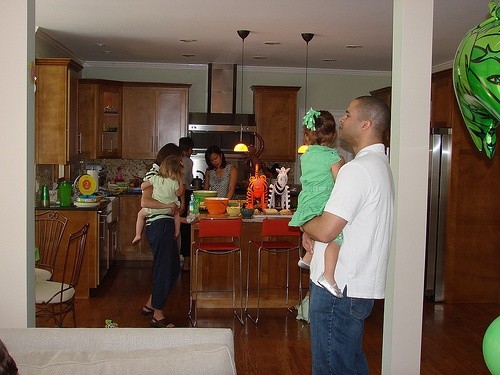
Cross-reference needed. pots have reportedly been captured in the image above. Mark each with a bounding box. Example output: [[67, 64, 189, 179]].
[[191, 176, 203, 189]]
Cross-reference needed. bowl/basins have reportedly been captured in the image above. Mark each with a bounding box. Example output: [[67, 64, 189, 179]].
[[205, 197, 228, 214], [193, 190, 218, 210], [240, 209, 255, 219], [226, 207, 242, 216]]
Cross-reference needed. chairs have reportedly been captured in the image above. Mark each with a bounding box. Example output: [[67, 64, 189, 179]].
[[33, 209, 90, 328]]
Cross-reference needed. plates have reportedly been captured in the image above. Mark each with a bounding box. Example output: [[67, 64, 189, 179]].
[[74, 201, 100, 207]]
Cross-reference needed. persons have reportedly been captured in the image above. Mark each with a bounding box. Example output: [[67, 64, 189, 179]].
[[299, 96, 394, 375], [204, 145, 239, 200], [288, 106, 345, 298], [132, 154, 183, 246], [132, 137, 193, 328]]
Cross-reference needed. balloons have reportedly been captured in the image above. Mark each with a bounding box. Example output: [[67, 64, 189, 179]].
[[453, 0, 500, 159], [481, 316, 500, 375]]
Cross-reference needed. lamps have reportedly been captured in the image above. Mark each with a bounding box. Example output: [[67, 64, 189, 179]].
[[297, 33, 315, 154], [234, 29, 250, 152]]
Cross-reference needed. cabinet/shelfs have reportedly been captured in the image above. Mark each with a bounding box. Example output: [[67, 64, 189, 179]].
[[369, 66, 500, 304], [32, 58, 302, 168], [34, 189, 152, 300], [189, 201, 308, 307]]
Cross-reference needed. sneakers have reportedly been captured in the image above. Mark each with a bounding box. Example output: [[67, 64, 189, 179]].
[[297, 256, 310, 270], [317, 274, 343, 299]]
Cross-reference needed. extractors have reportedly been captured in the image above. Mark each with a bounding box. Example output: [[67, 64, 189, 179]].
[[189, 63, 256, 155]]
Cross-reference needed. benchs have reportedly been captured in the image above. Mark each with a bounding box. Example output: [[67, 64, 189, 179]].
[[0, 327, 236, 375]]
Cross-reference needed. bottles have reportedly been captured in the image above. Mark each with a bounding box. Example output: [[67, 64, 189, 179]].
[[59, 181, 72, 207], [41, 186, 50, 207]]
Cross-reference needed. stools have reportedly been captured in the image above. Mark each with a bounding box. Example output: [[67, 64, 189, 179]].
[[246, 217, 303, 324], [190, 217, 245, 325]]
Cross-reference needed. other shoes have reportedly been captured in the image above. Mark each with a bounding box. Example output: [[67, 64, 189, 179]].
[[140, 305, 154, 315], [180, 262, 190, 271], [151, 317, 175, 327]]
